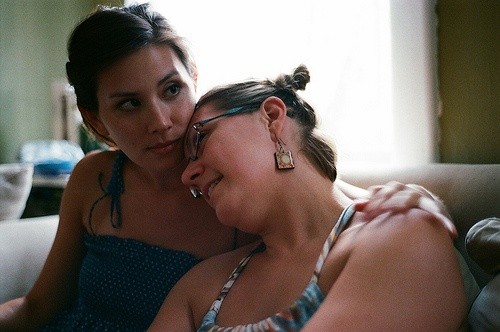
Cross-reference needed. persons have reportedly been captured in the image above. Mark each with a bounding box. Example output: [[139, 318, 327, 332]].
[[0, 1, 459, 332], [146, 64, 483, 332]]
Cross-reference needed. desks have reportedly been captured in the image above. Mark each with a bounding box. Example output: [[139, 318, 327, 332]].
[[31, 174, 68, 188]]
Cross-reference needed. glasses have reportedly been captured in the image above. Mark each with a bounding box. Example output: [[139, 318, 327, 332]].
[[181, 103, 294, 199]]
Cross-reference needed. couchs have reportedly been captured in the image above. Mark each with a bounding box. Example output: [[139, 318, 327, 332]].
[[0, 160, 500, 332]]
